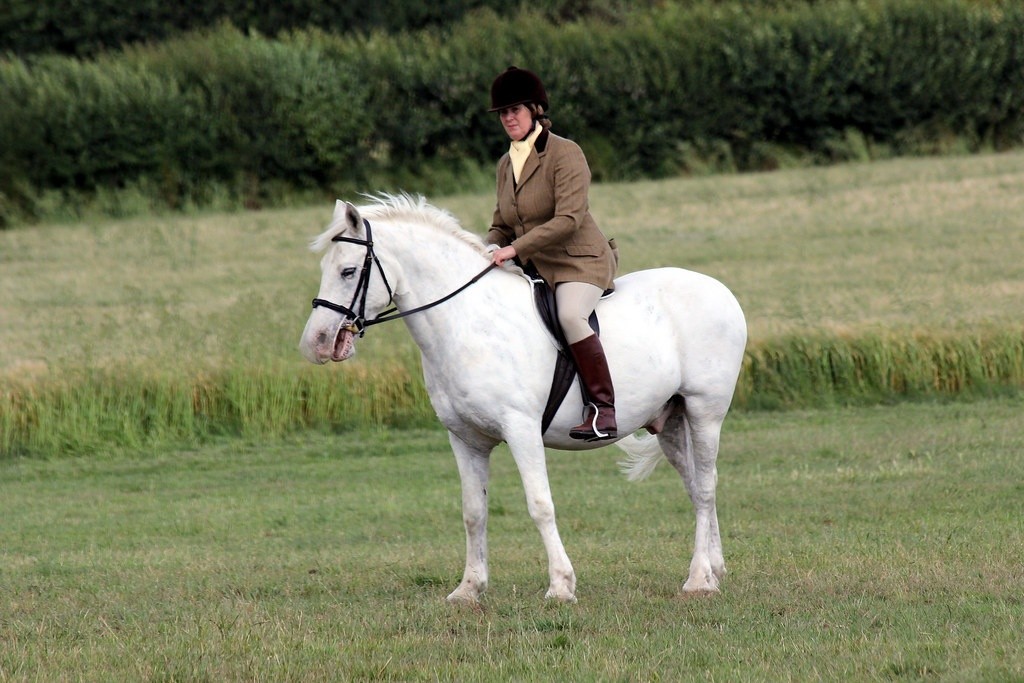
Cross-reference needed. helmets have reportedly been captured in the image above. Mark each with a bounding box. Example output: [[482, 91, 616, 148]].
[[486, 65, 548, 112]]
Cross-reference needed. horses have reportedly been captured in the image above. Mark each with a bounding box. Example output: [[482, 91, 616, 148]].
[[298, 189, 747, 606]]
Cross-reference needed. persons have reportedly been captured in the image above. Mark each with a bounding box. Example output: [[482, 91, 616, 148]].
[[482, 67, 620, 442]]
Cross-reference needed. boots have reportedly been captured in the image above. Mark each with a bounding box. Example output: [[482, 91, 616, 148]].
[[566, 331, 618, 441]]
